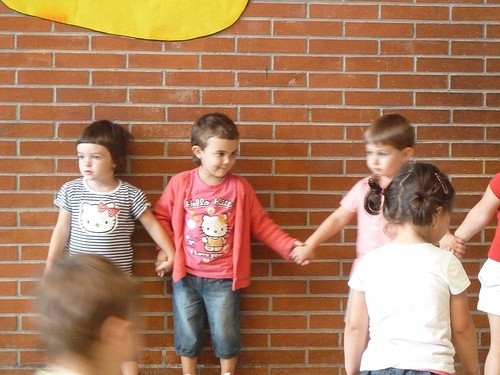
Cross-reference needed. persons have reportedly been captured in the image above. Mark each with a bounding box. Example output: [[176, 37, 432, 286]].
[[442, 171, 500, 375], [344, 163, 480, 375], [28, 254, 134, 375], [289, 112, 465, 328], [36, 119, 174, 375], [151, 112, 310, 375]]
